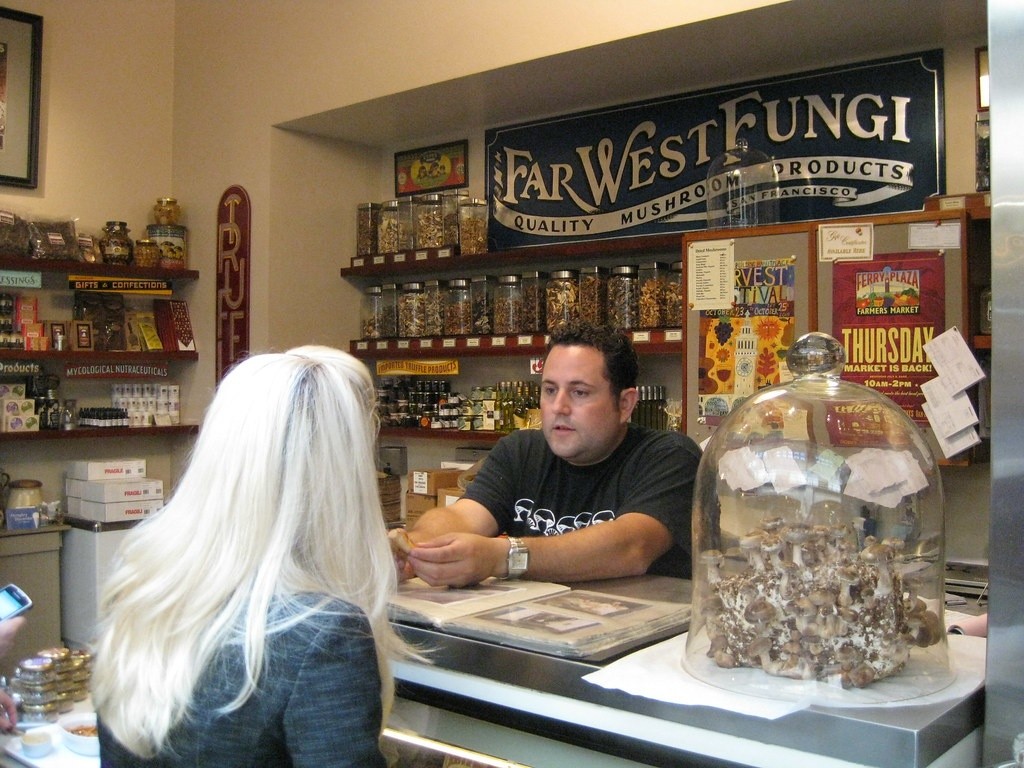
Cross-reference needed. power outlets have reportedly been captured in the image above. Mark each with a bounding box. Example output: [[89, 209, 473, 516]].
[[379, 446, 408, 475]]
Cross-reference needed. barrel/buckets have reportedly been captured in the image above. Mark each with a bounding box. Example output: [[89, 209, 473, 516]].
[[146, 223, 187, 270]]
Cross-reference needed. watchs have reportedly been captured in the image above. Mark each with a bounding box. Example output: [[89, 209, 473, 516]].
[[497, 536, 529, 581]]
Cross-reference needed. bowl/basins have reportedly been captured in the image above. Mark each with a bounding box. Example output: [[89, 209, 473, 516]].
[[57, 712, 99, 757]]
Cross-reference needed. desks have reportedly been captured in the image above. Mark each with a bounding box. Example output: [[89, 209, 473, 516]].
[[60, 516, 143, 651], [0, 689, 102, 768]]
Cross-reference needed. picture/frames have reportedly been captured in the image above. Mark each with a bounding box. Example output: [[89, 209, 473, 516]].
[[974, 45, 990, 111], [0, 6, 44, 189], [394, 139, 469, 197]]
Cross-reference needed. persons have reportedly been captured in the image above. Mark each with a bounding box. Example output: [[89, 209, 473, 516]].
[[86, 345, 439, 768], [0, 616, 26, 736], [389, 322, 721, 590]]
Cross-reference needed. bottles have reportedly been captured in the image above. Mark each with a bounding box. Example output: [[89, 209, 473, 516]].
[[377, 201, 397, 254], [377, 374, 541, 432], [459, 198, 487, 256], [78, 407, 129, 428], [630, 385, 667, 431], [415, 194, 442, 248], [99, 220, 133, 266], [397, 195, 419, 250], [153, 196, 181, 224], [442, 190, 470, 246], [134, 239, 159, 267], [356, 202, 381, 256], [5, 646, 94, 723], [6, 480, 43, 510], [360, 260, 684, 338], [53, 334, 67, 351]]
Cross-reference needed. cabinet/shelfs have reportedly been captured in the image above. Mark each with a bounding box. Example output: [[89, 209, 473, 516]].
[[0, 525, 72, 677], [341, 233, 685, 441], [0, 257, 199, 442]]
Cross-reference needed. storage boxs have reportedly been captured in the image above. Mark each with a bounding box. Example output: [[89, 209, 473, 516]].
[[12, 294, 50, 351], [0, 383, 39, 431], [39, 320, 94, 351], [66, 456, 164, 522], [5, 507, 40, 530], [406, 460, 477, 531]]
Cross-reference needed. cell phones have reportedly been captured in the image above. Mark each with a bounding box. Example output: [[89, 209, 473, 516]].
[[0, 583, 34, 622]]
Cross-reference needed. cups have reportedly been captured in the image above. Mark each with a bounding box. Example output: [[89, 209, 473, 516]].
[[110, 382, 180, 426]]
